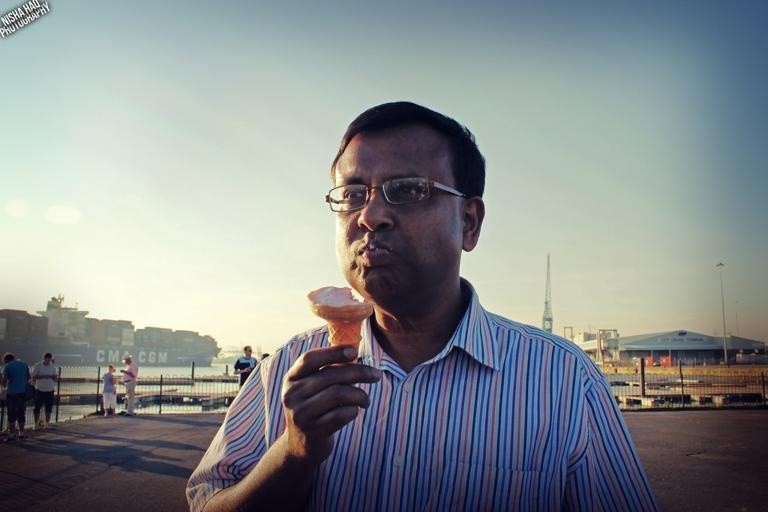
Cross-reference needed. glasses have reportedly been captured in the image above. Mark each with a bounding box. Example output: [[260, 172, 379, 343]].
[[325, 173, 463, 212]]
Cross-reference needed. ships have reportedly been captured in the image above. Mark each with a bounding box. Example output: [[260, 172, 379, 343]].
[[0, 297, 223, 368]]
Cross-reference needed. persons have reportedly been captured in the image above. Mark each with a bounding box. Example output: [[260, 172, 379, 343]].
[[119, 354, 138, 416], [100, 364, 119, 416], [186, 98, 658, 511], [2, 352, 32, 439], [32, 352, 57, 431], [233, 345, 257, 388]]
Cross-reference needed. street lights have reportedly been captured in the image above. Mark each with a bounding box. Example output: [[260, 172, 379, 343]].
[[716, 262, 728, 363]]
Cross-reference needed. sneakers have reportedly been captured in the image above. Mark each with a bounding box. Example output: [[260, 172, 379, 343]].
[[7, 425, 57, 440]]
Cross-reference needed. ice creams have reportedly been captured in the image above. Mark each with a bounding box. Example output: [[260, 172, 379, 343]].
[[306, 287, 374, 365]]
